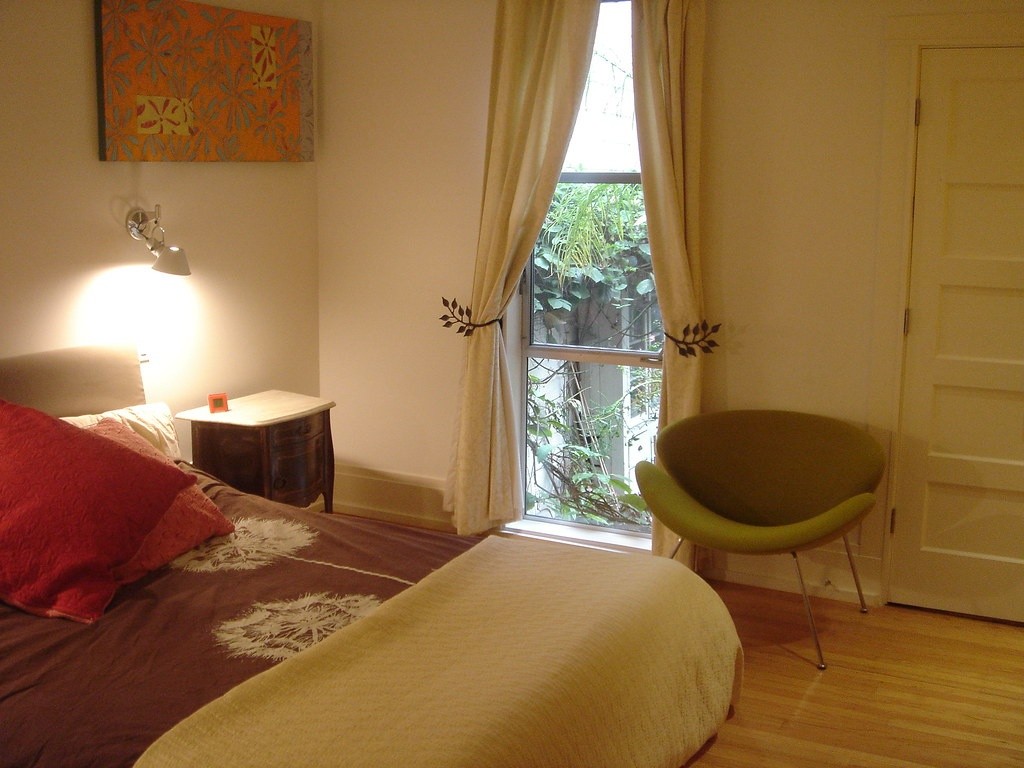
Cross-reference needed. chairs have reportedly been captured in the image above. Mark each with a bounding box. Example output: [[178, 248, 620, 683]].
[[634, 407, 887, 670]]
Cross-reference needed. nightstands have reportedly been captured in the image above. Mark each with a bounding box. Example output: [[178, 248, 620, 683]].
[[175, 388, 337, 515]]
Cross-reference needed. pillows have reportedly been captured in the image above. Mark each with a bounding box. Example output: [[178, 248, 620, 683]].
[[0, 397, 236, 623]]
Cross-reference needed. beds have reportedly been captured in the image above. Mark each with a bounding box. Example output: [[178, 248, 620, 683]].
[[1, 345, 746, 767]]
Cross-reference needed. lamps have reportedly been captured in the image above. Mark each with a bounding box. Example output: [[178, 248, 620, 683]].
[[125, 203, 194, 277]]
